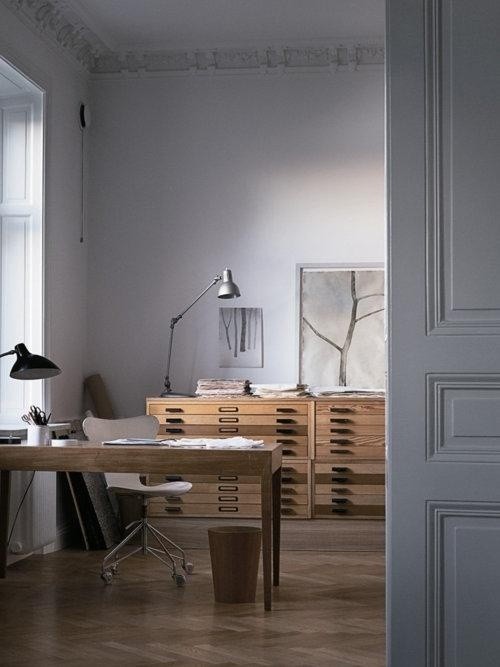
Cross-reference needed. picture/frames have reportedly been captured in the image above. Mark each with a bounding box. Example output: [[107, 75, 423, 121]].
[[294, 260, 388, 392]]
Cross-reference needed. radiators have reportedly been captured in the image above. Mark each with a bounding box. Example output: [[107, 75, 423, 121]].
[[13, 438, 56, 553]]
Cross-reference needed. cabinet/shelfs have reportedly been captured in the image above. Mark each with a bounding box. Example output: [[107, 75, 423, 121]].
[[311, 461, 385, 519], [145, 396, 311, 459], [145, 461, 313, 520], [314, 397, 386, 462]]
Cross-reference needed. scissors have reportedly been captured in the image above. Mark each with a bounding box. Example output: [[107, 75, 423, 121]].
[[30, 405, 41, 423], [31, 411, 46, 425], [22, 415, 31, 425]]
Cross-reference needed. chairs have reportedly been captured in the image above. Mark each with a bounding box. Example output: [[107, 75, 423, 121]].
[[82, 415, 194, 587]]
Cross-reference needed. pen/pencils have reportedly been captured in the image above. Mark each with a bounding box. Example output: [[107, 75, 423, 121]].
[[29, 412, 38, 425], [47, 413, 51, 423]]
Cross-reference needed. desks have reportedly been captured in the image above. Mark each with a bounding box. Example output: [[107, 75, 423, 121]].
[[0, 440, 282, 610]]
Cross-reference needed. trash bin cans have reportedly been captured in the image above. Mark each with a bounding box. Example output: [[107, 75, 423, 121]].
[[208, 526, 262, 603]]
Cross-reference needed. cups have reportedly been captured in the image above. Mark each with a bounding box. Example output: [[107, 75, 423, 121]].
[[27, 425, 51, 446]]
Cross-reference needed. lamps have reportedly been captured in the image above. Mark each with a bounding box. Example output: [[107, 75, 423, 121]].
[[163, 267, 241, 400], [0, 344, 63, 380]]
